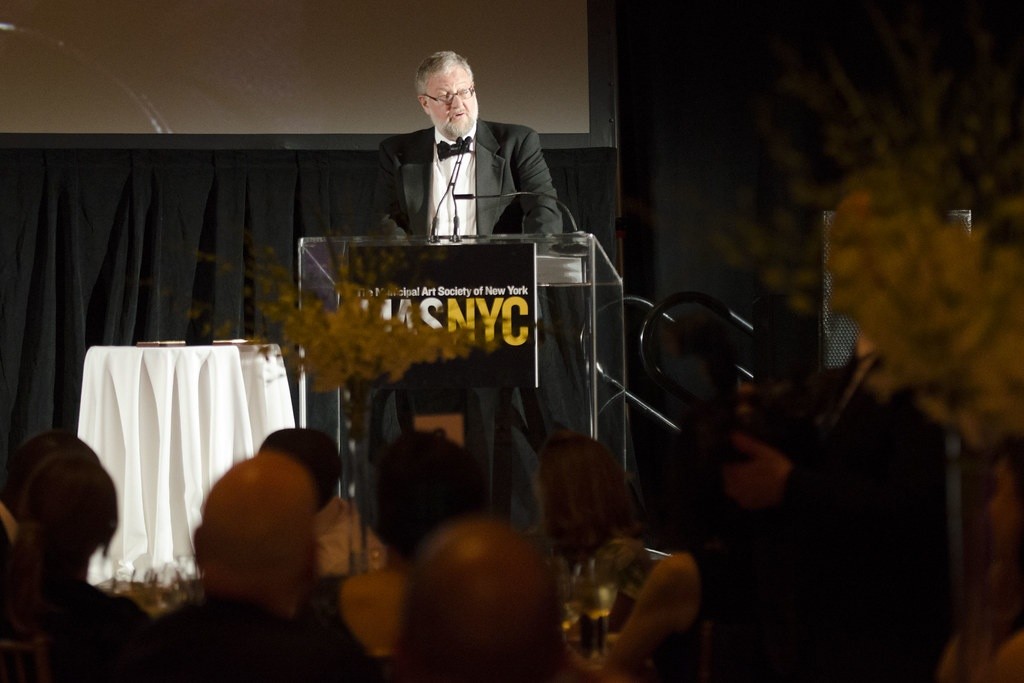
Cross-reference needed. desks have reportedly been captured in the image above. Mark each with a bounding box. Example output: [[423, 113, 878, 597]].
[[76, 343, 295, 586]]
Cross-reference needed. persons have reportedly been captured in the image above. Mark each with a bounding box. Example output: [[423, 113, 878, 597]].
[[0, 366, 1024, 683], [371, 52, 564, 235]]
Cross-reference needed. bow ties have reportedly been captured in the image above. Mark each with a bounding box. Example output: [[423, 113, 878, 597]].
[[437, 136, 472, 161]]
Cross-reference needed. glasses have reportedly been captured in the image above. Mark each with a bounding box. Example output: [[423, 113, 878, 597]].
[[422, 84, 476, 105]]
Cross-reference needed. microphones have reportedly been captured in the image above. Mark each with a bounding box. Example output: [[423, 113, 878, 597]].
[[452, 136, 472, 242], [430, 137, 464, 243], [453, 191, 578, 232]]
